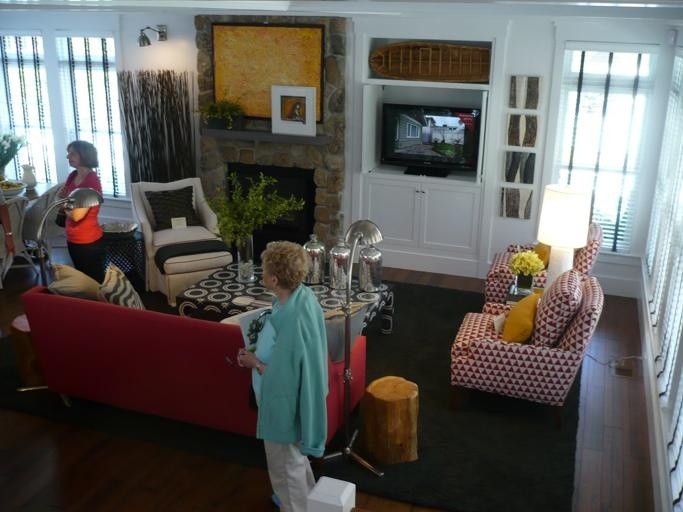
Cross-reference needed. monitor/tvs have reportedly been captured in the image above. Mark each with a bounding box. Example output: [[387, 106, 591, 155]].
[[380, 102, 481, 178]]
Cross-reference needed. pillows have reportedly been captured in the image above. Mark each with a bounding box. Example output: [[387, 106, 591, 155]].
[[502, 295, 540, 346], [145, 186, 197, 228], [530, 271, 582, 346], [48, 261, 101, 302], [97, 263, 143, 312]]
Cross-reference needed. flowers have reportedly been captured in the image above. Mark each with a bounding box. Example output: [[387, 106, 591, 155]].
[[505, 249, 545, 279], [0, 131, 26, 179]]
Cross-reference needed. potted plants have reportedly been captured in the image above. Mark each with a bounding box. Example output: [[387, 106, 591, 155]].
[[208, 174, 305, 283]]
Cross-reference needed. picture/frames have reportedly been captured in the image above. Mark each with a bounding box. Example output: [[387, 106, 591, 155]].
[[270, 84, 316, 137]]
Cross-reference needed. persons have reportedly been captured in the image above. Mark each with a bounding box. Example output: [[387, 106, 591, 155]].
[[53, 140, 106, 286], [236, 240, 331, 512]]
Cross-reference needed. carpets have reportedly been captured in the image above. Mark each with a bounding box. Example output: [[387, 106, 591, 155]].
[[0, 279, 583, 512]]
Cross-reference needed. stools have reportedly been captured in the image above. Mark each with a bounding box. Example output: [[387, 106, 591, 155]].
[[366, 376, 419, 465]]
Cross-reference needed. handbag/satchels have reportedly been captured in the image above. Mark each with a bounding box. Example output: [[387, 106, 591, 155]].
[[55, 208, 67, 228]]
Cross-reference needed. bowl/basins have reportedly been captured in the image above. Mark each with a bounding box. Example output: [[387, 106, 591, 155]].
[[0, 180, 27, 198]]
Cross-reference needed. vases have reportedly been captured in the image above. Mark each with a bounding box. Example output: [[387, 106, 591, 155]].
[[515, 272, 534, 288]]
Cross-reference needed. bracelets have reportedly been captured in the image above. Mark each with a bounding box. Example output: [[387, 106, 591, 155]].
[[255, 360, 262, 376]]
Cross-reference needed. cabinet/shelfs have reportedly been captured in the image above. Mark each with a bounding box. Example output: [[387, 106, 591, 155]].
[[360, 33, 494, 275]]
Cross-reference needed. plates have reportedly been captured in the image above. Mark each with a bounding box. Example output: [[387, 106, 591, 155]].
[[232, 296, 255, 307]]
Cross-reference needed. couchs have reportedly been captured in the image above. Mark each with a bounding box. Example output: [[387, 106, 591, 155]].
[[130, 178, 235, 305], [451, 271, 605, 407], [485, 223, 602, 304], [20, 284, 367, 450]]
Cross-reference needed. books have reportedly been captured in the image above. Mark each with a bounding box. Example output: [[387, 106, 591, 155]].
[[237, 307, 266, 354]]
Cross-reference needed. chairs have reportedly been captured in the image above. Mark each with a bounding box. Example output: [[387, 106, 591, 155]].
[[22, 183, 69, 268], [0, 197, 38, 287]]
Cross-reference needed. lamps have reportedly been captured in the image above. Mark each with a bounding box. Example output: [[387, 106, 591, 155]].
[[537, 183, 594, 293], [321, 221, 385, 476], [138, 25, 168, 47], [36, 188, 103, 287]]
